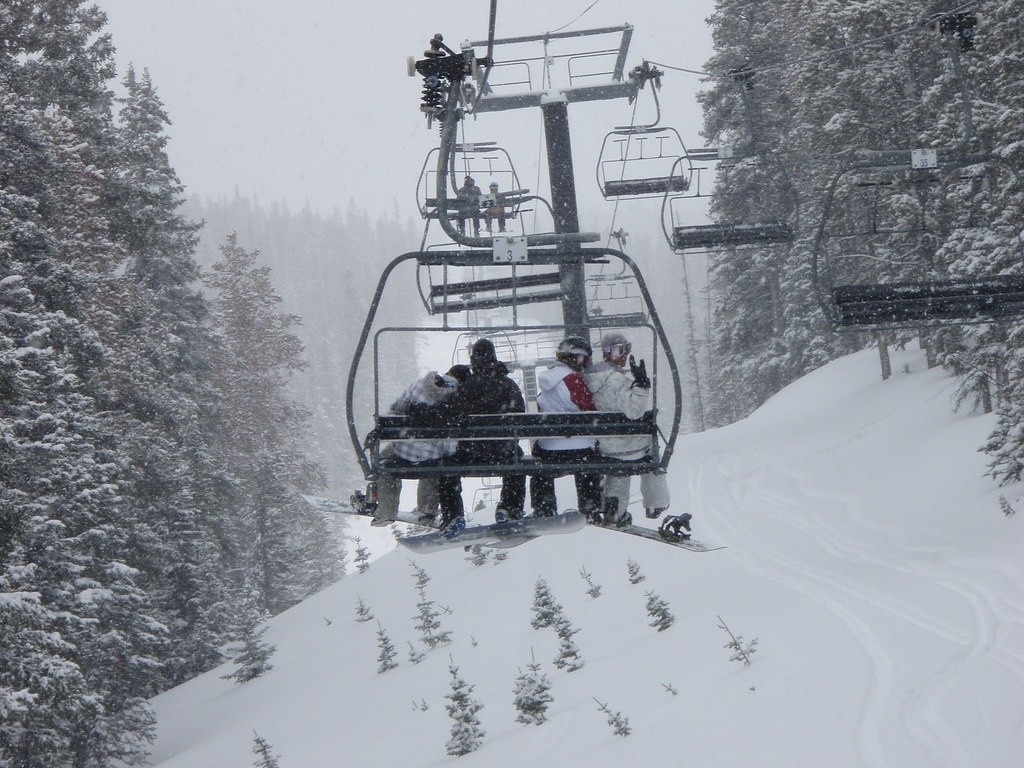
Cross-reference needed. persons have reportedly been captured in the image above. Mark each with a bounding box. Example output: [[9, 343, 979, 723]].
[[581, 332, 670, 526], [370, 363, 470, 527], [435, 339, 526, 538], [482, 182, 506, 231], [457, 177, 482, 238], [526, 336, 603, 525]]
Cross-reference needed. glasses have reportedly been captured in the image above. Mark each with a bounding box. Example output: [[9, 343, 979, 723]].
[[613, 342, 632, 358]]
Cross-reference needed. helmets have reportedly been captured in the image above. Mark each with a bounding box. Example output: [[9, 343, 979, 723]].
[[602, 332, 627, 358], [444, 365, 472, 384], [489, 182, 498, 189], [471, 340, 496, 359], [556, 336, 592, 372]]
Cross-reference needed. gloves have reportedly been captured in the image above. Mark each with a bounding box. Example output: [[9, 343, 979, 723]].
[[629, 354, 651, 389]]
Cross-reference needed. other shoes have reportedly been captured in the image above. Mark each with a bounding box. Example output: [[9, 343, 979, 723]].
[[473, 230, 480, 237], [586, 511, 602, 525], [500, 226, 506, 232], [371, 516, 395, 528], [419, 512, 436, 523], [459, 230, 465, 235], [645, 504, 671, 519], [487, 226, 493, 232], [495, 508, 516, 522], [604, 511, 632, 527], [528, 508, 557, 518], [439, 517, 466, 539]]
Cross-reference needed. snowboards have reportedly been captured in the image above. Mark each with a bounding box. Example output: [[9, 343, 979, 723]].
[[587, 518, 729, 553], [396, 511, 588, 555], [300, 493, 442, 529]]
